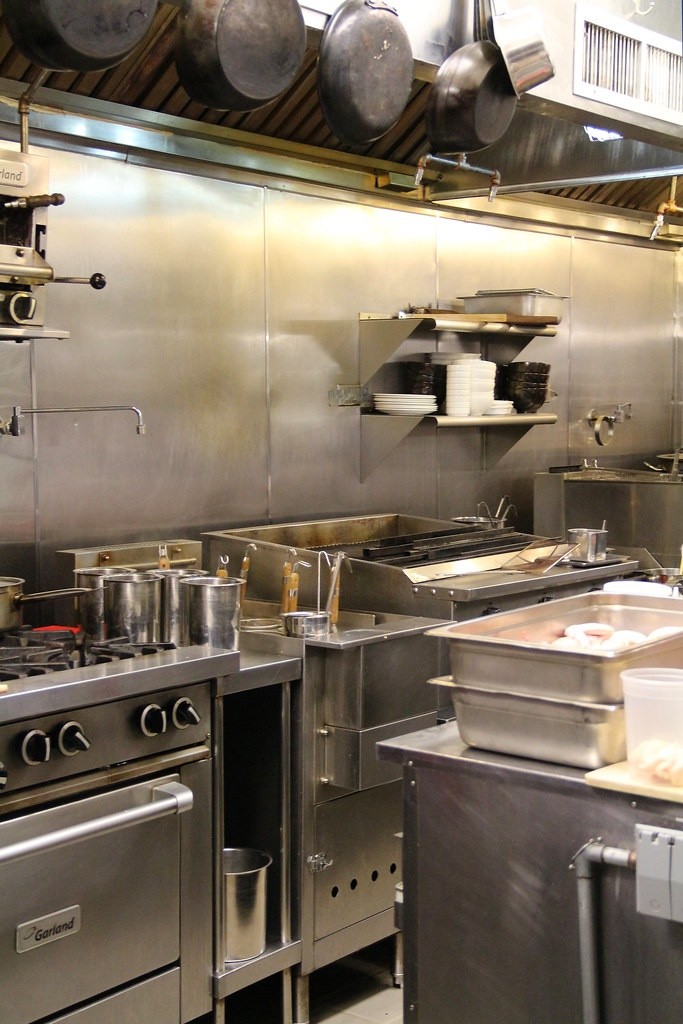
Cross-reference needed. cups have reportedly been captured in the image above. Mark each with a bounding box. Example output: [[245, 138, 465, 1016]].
[[567, 528, 608, 563]]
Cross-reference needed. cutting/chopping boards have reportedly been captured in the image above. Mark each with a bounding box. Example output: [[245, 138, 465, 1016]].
[[584, 759, 683, 804]]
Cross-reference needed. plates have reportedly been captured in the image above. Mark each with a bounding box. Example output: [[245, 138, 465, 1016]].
[[372, 394, 438, 415], [603, 581, 672, 596], [484, 400, 514, 415]]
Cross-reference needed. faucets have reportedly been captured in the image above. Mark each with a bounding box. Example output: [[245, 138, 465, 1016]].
[[615, 400, 633, 423], [3, 404, 147, 436]]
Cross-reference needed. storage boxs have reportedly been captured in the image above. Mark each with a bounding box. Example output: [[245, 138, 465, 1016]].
[[422, 587, 683, 767], [464, 296, 564, 321]]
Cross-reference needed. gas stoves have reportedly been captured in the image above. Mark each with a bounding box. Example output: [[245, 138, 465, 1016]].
[[0, 624, 241, 794]]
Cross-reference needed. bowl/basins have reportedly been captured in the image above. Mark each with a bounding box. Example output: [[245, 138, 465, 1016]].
[[656, 454, 683, 474], [405, 352, 497, 416], [619, 668, 683, 787], [643, 568, 683, 588], [279, 610, 332, 638], [494, 362, 552, 414]]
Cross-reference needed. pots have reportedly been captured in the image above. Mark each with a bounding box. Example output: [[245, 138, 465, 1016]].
[[315, 0, 414, 148], [425, 40, 518, 153], [0, 0, 159, 73], [493, 0, 556, 99], [174, 0, 306, 111], [0, 576, 99, 632]]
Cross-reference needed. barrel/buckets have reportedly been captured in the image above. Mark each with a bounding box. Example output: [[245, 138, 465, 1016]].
[[145, 569, 211, 647], [102, 574, 165, 644], [178, 577, 247, 650], [223, 848, 273, 962], [72, 567, 137, 641]]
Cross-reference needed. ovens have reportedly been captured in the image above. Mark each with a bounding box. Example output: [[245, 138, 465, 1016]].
[[0, 745, 212, 1024]]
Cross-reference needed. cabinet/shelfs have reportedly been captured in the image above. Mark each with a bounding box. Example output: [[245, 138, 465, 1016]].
[[362, 309, 562, 429]]
